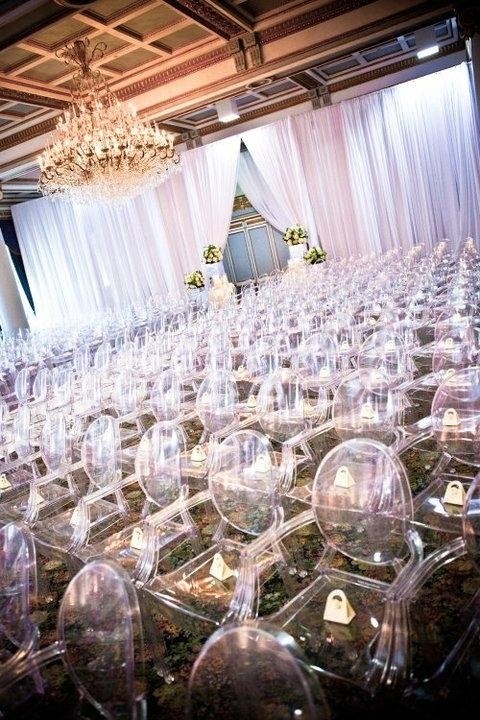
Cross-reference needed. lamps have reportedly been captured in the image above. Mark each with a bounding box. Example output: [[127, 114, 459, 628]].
[[216, 100, 240, 123], [416, 25, 440, 60], [33, 36, 186, 208]]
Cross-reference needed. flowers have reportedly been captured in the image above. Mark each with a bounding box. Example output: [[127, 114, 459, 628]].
[[282, 223, 311, 246]]
